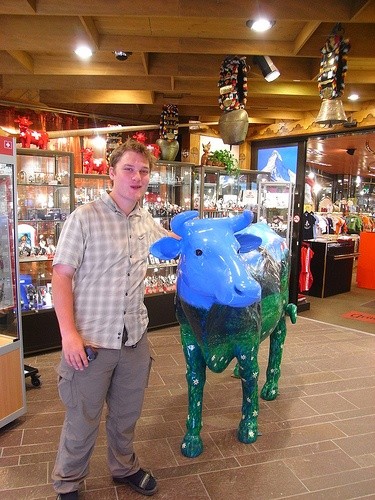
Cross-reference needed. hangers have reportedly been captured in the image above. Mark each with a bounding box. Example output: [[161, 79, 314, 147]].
[[304, 209, 314, 216]]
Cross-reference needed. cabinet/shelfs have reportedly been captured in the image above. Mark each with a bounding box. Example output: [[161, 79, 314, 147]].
[[305, 239, 355, 298], [0, 148, 294, 431]]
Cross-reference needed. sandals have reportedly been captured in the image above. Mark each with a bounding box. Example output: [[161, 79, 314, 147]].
[[115, 469, 158, 496], [56, 491, 78, 500]]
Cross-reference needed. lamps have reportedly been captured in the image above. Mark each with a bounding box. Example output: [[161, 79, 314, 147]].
[[252, 55, 280, 83], [114, 51, 132, 61], [347, 149, 357, 155]]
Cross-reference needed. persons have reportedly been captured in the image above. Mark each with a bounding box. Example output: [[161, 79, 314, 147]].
[[51, 142, 181, 500]]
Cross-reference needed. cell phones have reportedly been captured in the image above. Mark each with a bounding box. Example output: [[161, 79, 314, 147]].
[[82, 344, 97, 366]]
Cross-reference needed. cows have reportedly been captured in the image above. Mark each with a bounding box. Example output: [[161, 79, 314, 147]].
[[148, 207, 298, 461]]
